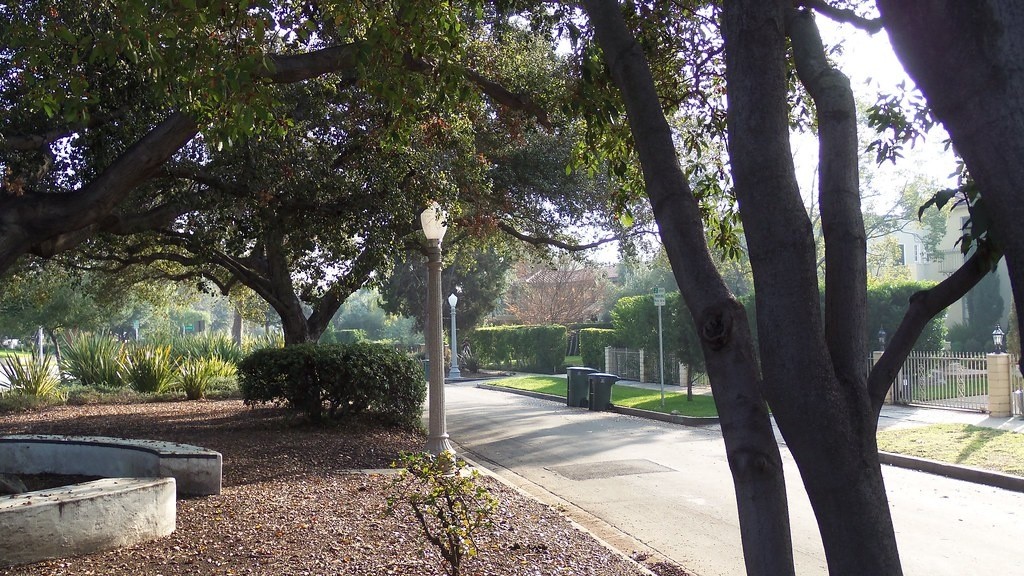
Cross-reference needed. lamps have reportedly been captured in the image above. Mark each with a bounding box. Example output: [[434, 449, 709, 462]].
[[876, 323, 888, 351], [991, 323, 1005, 354]]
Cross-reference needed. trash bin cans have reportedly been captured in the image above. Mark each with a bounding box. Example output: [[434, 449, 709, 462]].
[[565, 366, 599, 407], [902, 374, 912, 402], [587, 373, 621, 412]]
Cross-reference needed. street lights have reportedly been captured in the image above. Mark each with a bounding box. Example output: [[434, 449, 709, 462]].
[[420, 201, 456, 474], [447, 292, 461, 379]]
